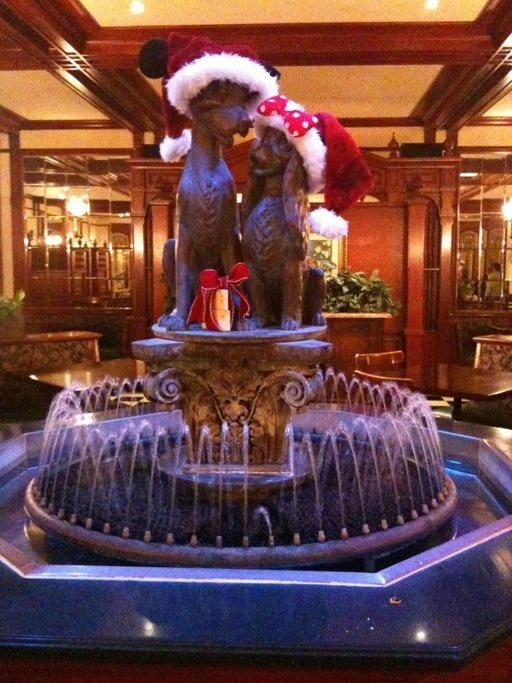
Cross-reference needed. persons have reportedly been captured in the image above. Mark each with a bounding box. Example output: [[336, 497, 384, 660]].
[[485, 262, 503, 300]]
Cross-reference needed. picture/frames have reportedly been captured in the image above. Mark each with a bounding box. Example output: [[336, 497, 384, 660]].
[[299, 226, 348, 280]]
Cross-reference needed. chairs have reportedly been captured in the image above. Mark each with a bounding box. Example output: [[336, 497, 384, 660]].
[[353, 349, 411, 408]]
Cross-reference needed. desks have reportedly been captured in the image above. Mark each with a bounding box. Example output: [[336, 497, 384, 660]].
[[31, 357, 147, 407], [396, 362, 511, 420]]
